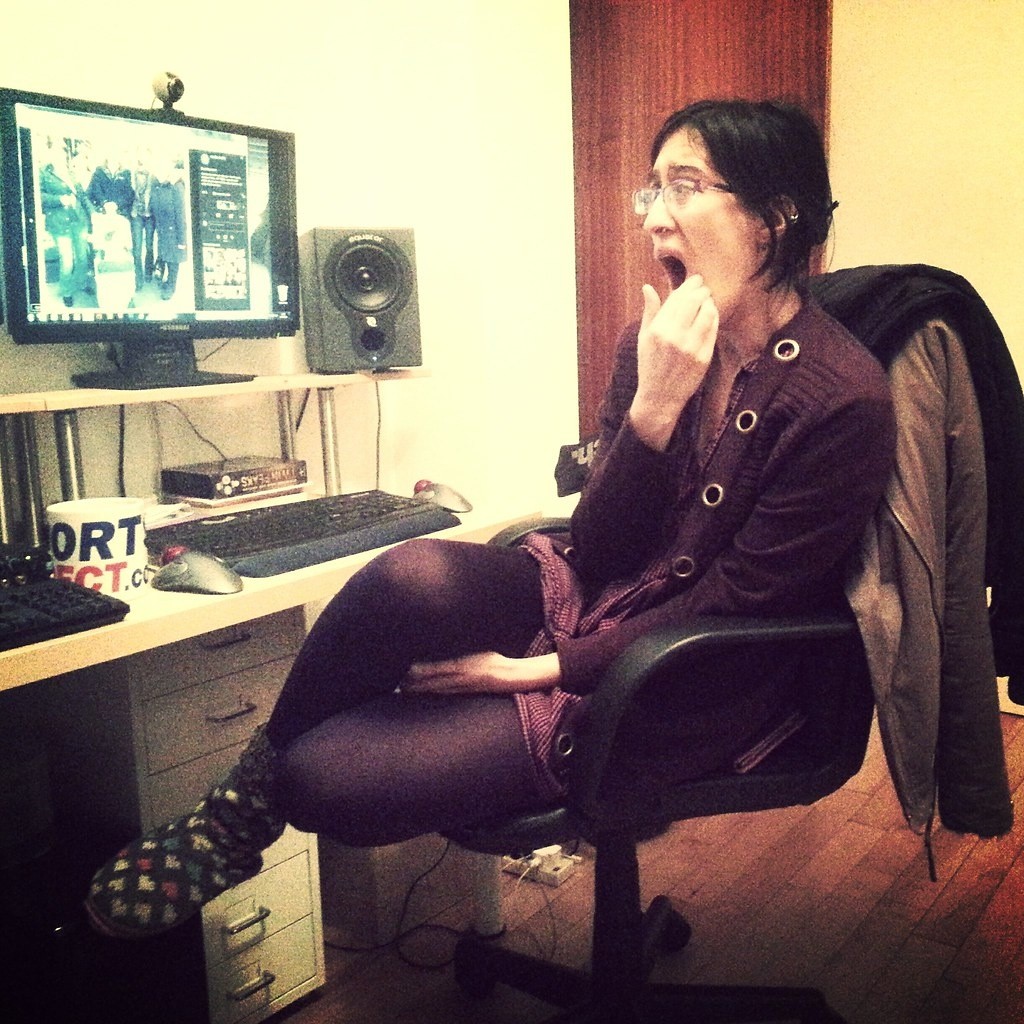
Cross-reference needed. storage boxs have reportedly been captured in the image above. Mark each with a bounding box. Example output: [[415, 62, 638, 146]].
[[160, 455, 306, 501]]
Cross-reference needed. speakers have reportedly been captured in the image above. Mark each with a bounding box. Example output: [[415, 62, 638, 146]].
[[297, 227, 421, 373]]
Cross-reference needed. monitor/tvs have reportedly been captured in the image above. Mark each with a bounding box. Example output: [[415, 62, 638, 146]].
[[1, 89, 302, 389]]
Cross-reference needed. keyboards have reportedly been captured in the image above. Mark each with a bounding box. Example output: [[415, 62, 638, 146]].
[[145, 490, 461, 579], [0, 578, 130, 653]]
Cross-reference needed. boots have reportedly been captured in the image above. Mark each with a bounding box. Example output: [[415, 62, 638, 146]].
[[83, 718, 298, 942]]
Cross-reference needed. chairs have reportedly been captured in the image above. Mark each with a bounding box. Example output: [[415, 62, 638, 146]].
[[432, 265, 990, 1024]]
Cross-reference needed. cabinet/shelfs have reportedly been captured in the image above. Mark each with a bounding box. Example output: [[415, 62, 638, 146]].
[[37, 605, 331, 1024]]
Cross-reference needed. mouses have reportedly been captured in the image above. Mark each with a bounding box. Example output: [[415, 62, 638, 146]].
[[410, 480, 473, 513], [150, 545, 243, 594]]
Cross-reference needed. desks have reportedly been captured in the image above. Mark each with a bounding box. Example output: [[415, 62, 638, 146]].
[[0, 368, 547, 701]]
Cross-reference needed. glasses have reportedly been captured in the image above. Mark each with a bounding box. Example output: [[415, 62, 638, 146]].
[[631, 178, 739, 215]]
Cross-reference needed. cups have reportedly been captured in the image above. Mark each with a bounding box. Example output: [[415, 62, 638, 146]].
[[45, 497, 147, 596]]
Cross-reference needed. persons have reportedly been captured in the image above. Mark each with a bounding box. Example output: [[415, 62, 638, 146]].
[[80, 98, 898, 942], [34, 132, 189, 309]]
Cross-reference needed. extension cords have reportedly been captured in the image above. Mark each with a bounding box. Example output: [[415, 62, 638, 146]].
[[501, 854, 576, 887]]
